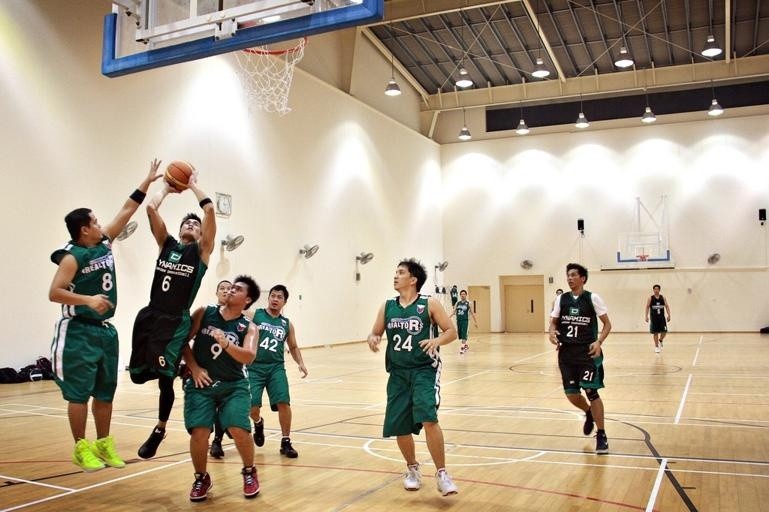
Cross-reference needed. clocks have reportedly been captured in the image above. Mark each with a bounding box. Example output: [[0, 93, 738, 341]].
[[216, 192, 232, 216]]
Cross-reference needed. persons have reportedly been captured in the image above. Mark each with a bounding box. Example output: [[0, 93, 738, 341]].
[[644, 285, 671, 353], [48, 158, 162, 472], [448, 290, 478, 353], [244, 284, 309, 459], [182, 274, 260, 502], [548, 263, 612, 453], [551, 288, 563, 311], [129, 171, 218, 459], [367, 256, 458, 497]]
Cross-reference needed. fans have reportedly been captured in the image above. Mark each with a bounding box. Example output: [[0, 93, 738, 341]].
[[222, 235, 244, 251], [356, 253, 374, 264], [299, 245, 319, 259], [435, 261, 448, 272], [520, 260, 533, 269], [708, 253, 720, 264], [117, 221, 138, 241]]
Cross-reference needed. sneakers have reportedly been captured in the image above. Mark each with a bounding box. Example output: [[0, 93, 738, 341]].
[[138, 417, 298, 501], [435, 472, 458, 497], [460, 347, 466, 354], [655, 346, 660, 353], [72, 437, 106, 471], [659, 339, 663, 348], [583, 407, 594, 435], [465, 344, 469, 350], [403, 462, 421, 490], [593, 432, 608, 454], [92, 436, 125, 468]]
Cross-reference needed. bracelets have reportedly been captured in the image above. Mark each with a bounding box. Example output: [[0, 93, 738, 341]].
[[597, 339, 602, 345], [199, 198, 212, 208], [129, 188, 146, 205]]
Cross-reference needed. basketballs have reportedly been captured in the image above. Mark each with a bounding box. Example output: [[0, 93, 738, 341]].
[[166, 161, 192, 190]]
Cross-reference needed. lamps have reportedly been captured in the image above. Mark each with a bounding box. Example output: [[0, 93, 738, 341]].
[[708, 80, 724, 115], [455, 11, 473, 87], [458, 109, 472, 139], [384, 0, 401, 95], [575, 93, 589, 128], [531, 0, 550, 78], [516, 103, 530, 134], [700, 0, 722, 57], [642, 89, 656, 122], [615, 0, 634, 67]]
